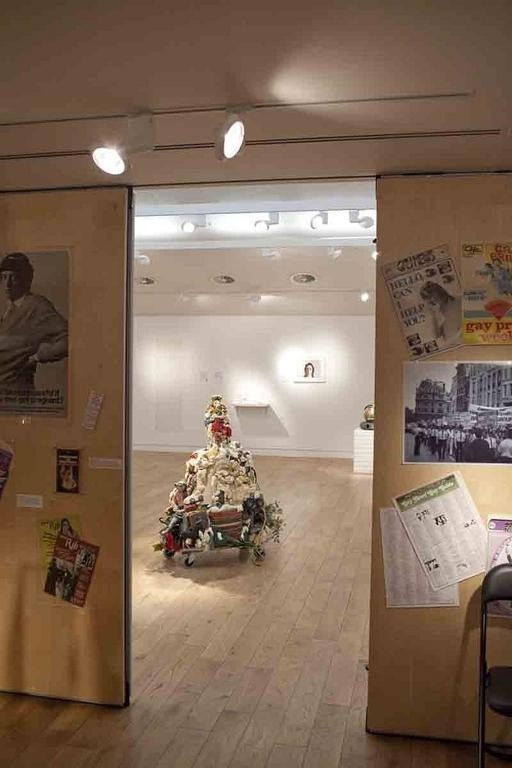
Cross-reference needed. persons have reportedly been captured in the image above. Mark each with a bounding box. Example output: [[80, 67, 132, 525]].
[[419, 281, 461, 346], [411, 422, 512, 463], [303, 363, 315, 378], [0, 253, 67, 405], [58, 518, 80, 540]]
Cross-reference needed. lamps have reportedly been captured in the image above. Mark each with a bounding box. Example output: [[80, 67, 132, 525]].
[[91, 99, 255, 175], [135, 206, 376, 265]]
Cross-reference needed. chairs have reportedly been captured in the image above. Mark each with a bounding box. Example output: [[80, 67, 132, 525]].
[[477, 563, 512, 768]]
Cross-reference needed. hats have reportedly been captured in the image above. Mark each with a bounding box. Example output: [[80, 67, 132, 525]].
[[0, 253, 32, 271]]
